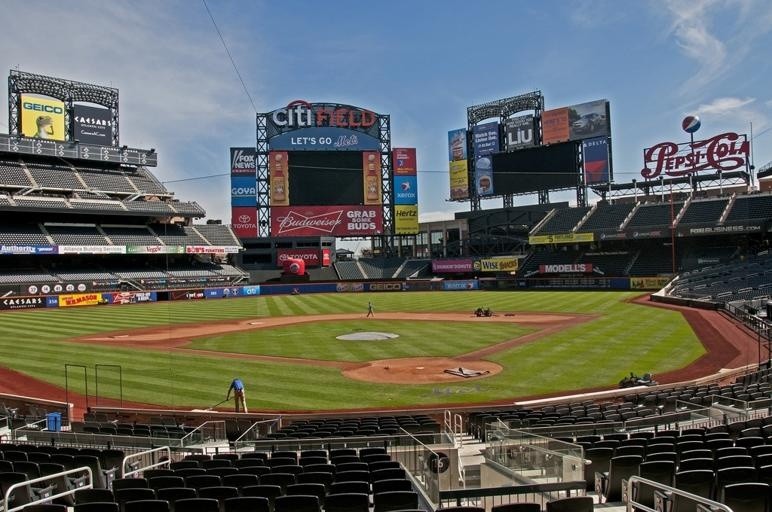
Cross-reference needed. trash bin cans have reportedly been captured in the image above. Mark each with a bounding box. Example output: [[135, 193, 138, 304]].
[[45, 412, 62, 432]]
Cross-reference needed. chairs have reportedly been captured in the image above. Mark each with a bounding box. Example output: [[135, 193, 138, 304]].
[[0, 363, 770, 511]]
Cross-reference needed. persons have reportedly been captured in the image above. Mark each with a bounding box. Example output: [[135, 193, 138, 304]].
[[477, 307, 493, 317], [227, 378, 247, 413], [366, 302, 375, 317]]
